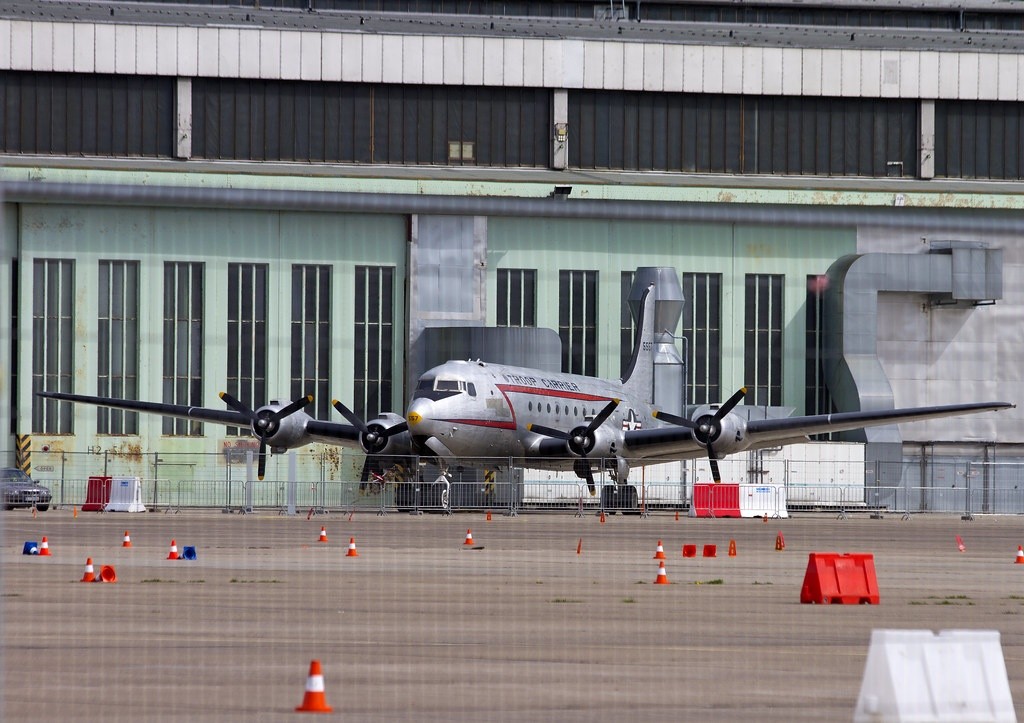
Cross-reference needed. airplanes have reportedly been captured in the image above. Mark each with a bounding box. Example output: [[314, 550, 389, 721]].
[[34, 285, 1016, 514]]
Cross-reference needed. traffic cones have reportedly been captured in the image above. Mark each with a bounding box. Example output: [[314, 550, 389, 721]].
[[1014, 546, 1024, 564], [463, 528, 475, 545], [345, 537, 359, 556], [682, 545, 717, 557], [293, 659, 334, 713], [80, 558, 119, 583], [122, 531, 132, 547], [22, 536, 51, 556], [166, 540, 198, 560], [653, 541, 667, 559], [318, 527, 328, 541], [654, 562, 670, 584]]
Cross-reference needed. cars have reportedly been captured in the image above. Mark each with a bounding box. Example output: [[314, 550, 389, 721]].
[[0, 467, 53, 512]]
